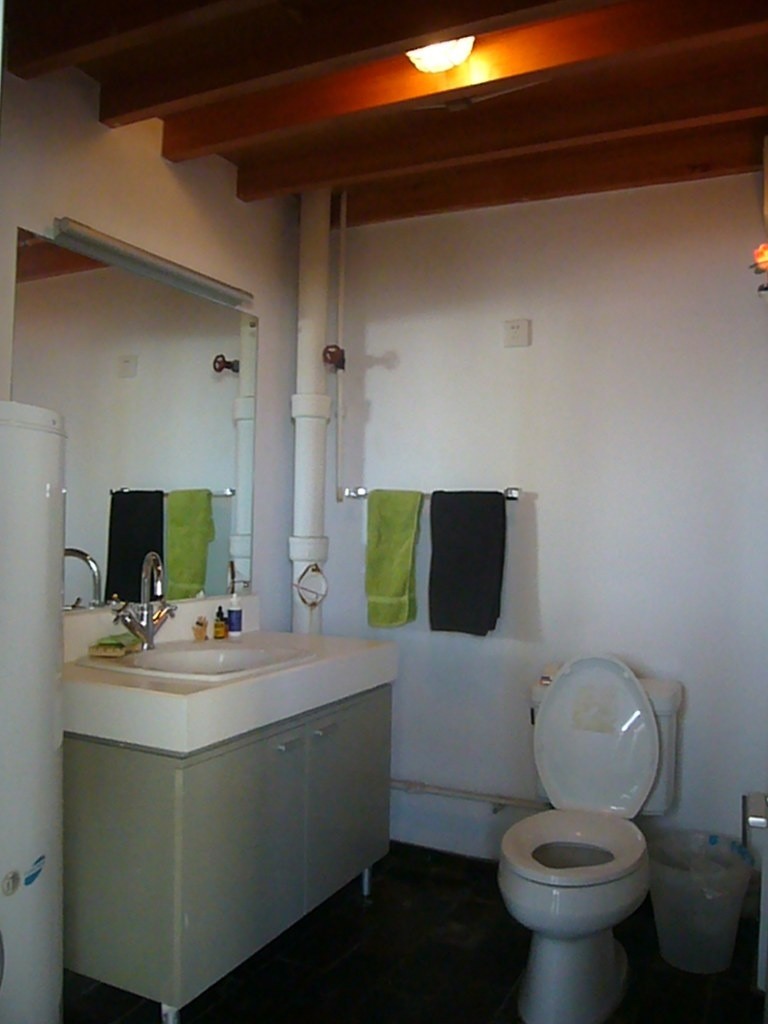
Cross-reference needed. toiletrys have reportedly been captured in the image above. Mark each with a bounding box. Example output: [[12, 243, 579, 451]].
[[226, 591, 244, 638], [211, 603, 228, 639]]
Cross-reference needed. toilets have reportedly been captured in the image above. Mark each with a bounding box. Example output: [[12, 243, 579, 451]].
[[497, 648, 686, 1024]]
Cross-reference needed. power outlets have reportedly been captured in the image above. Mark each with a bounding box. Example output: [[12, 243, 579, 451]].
[[502, 319, 534, 348]]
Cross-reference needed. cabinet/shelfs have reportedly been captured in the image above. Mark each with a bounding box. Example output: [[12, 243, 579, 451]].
[[65, 684, 394, 1024]]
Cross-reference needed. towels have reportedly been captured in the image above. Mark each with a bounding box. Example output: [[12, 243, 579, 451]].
[[164, 487, 216, 602], [361, 486, 425, 631], [102, 489, 166, 606], [425, 488, 512, 636]]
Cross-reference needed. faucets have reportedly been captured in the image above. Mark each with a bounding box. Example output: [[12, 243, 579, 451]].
[[64, 547, 104, 610], [108, 551, 178, 654]]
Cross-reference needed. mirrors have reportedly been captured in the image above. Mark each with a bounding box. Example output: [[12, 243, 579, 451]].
[[12, 224, 260, 615]]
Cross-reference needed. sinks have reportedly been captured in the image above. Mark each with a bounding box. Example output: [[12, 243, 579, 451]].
[[76, 634, 318, 683]]
